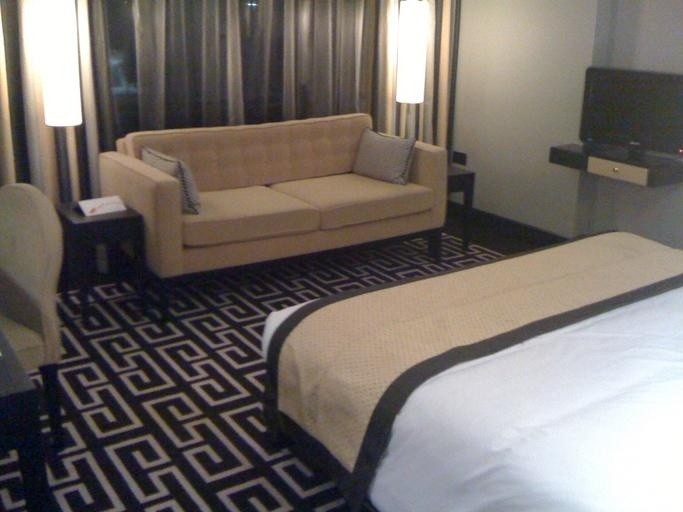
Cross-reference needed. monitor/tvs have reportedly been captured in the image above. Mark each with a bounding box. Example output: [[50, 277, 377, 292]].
[[579, 68, 683, 155]]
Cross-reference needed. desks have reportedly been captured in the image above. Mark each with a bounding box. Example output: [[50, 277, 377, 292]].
[[0, 320, 51, 512], [431, 163, 476, 261], [55, 197, 149, 327]]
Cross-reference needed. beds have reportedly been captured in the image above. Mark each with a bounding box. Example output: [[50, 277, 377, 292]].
[[259, 228, 682, 512]]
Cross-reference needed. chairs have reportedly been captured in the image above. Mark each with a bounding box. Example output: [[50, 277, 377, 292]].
[[0, 179, 64, 447]]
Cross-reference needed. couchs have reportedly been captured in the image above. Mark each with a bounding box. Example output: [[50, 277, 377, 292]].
[[98, 111, 448, 312]]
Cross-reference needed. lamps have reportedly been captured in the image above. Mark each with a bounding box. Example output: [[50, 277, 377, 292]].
[[33, 0, 84, 203], [393, 1, 432, 142]]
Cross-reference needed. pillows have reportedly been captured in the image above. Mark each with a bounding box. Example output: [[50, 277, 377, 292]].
[[351, 125, 417, 187], [140, 145, 201, 216]]
